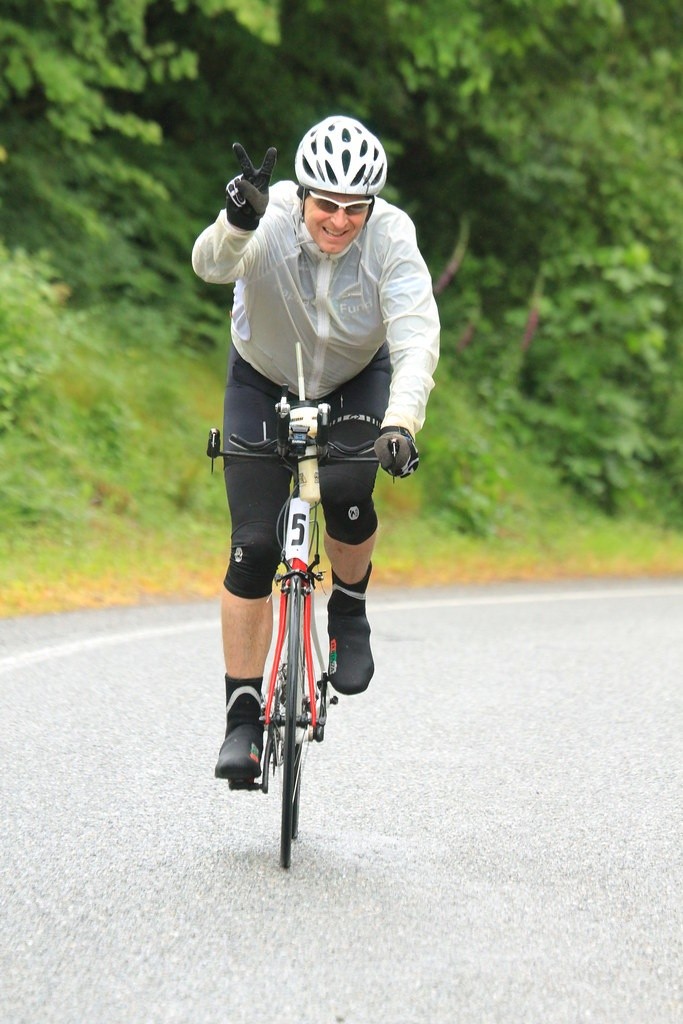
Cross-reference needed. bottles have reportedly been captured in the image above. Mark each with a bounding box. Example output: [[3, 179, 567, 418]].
[[288, 399, 320, 504]]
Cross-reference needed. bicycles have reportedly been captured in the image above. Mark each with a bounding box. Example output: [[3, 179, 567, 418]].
[[206, 340, 399, 871]]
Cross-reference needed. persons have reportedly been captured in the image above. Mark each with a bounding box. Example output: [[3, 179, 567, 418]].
[[191, 116, 440, 779]]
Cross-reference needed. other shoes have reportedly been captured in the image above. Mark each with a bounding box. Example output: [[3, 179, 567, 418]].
[[327, 601, 375, 695], [215, 712, 264, 781]]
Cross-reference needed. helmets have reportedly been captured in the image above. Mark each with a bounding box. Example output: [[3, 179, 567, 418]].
[[293, 115, 386, 200]]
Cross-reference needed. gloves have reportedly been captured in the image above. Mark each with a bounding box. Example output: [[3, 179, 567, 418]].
[[224, 142, 277, 231], [375, 427, 419, 480]]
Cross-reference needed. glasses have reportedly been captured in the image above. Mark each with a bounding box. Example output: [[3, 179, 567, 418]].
[[310, 189, 373, 216]]
